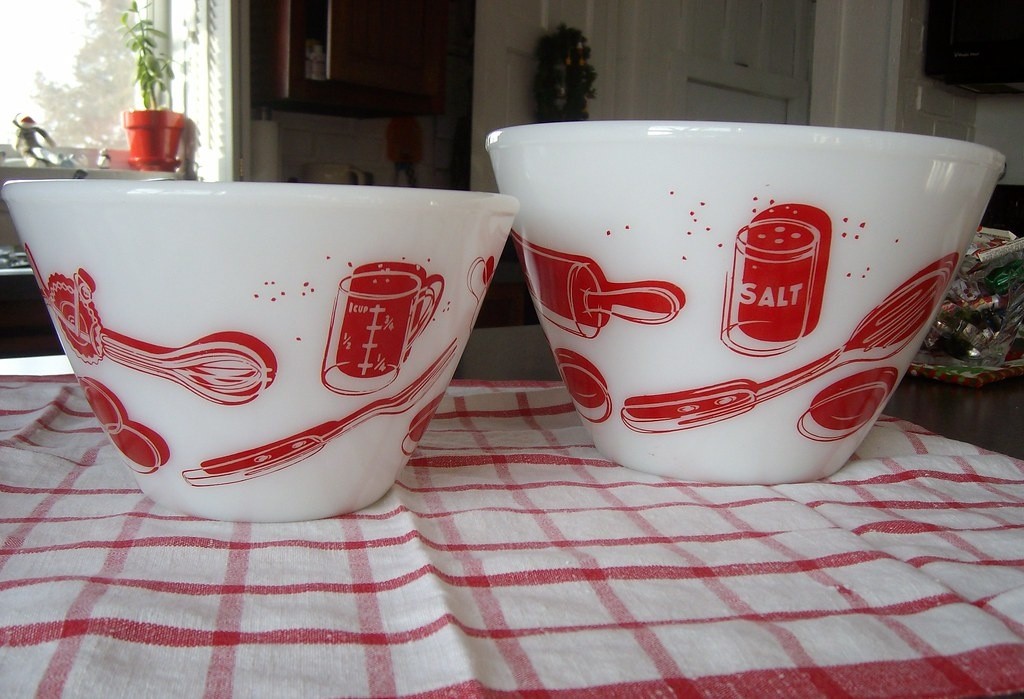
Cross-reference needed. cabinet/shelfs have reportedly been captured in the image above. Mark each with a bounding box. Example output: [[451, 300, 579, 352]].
[[251, 1, 448, 117]]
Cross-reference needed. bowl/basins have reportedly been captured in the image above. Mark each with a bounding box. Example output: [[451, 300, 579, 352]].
[[487, 119, 1007, 485], [2, 178, 520, 522]]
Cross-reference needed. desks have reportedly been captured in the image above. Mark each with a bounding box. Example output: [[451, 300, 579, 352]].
[[1, 355, 1024, 699]]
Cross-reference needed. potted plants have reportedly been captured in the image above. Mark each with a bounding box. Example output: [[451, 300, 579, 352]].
[[120, 0, 184, 172]]
